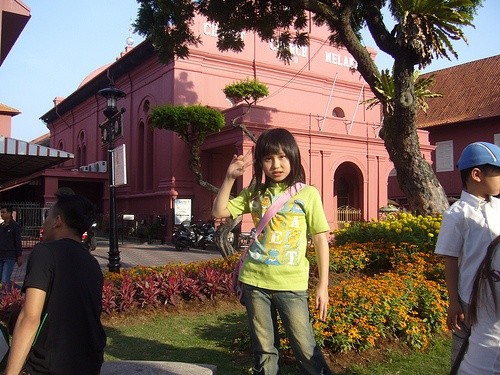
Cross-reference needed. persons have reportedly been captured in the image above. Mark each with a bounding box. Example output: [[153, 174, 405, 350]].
[[212, 127, 332, 375], [0, 189, 107, 375], [434, 142, 500, 375]]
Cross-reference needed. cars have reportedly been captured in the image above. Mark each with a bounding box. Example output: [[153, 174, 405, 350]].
[[39, 222, 97, 250]]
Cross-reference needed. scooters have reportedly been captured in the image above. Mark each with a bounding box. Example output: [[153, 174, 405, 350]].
[[171, 217, 216, 251], [235, 228, 256, 251]]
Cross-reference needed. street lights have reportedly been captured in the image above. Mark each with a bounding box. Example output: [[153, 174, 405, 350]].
[[96, 81, 127, 273]]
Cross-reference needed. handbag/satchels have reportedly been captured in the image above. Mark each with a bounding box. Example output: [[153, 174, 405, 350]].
[[231, 257, 245, 307]]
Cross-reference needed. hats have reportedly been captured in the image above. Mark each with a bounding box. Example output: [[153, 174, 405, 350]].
[[457, 141, 500, 173]]
